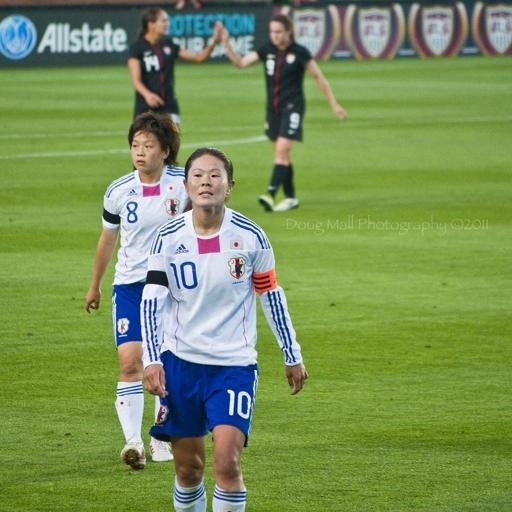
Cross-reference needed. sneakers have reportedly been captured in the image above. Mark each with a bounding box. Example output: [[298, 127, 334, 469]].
[[148, 436, 175, 463], [119, 435, 146, 471], [257, 194, 299, 213]]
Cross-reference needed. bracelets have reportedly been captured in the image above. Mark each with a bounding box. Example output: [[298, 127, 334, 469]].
[[207, 37, 215, 47]]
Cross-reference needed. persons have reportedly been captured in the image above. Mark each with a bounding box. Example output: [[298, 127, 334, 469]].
[[173, 0, 203, 11], [83, 110, 194, 473], [139, 147, 308, 511], [215, 13, 347, 216], [128, 5, 223, 173]]
